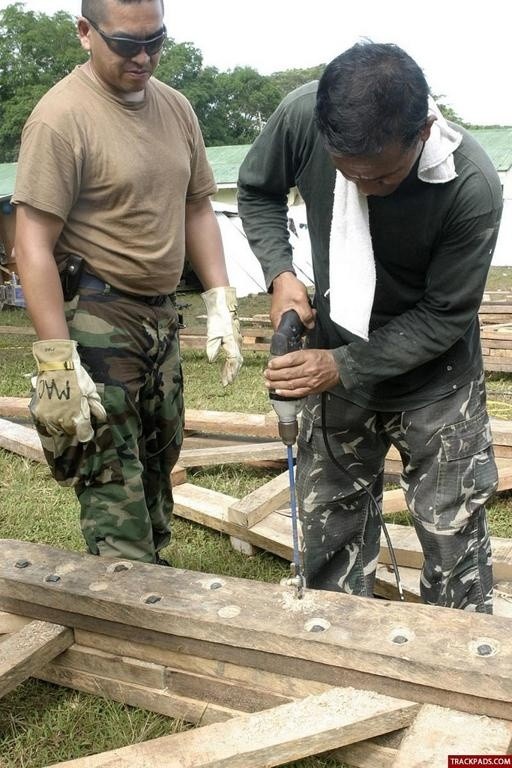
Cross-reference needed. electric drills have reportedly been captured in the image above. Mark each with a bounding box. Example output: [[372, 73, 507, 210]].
[[268, 308, 314, 600]]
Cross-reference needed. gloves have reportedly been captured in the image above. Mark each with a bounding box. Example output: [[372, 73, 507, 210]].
[[201, 285, 245, 387], [29, 339, 107, 443]]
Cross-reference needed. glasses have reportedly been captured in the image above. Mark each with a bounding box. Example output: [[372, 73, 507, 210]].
[[88, 17, 168, 58]]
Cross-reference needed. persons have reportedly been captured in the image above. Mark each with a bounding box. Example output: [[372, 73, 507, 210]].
[[237, 36, 504, 612], [11, 0, 244, 566]]
